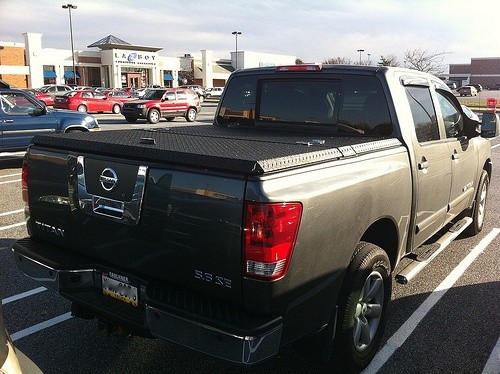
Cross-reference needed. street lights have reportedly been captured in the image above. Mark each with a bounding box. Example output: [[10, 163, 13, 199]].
[[232, 31, 242, 71], [62, 4, 78, 88], [357, 49, 364, 65]]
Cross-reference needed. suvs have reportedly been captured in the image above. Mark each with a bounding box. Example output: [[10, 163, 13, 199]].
[[121, 87, 203, 124]]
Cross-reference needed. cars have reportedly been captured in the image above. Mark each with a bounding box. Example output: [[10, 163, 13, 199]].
[[459, 84, 483, 96], [15, 83, 225, 114], [0, 88, 101, 162]]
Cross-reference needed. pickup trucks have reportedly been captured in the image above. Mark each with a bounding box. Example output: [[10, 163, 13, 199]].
[[10, 62, 492, 373]]
